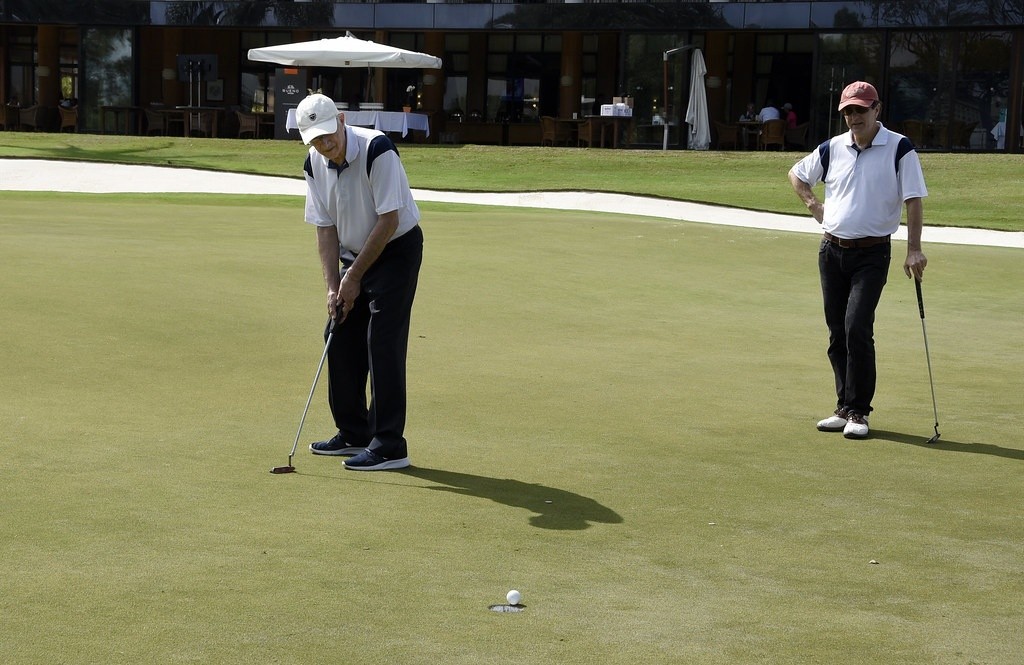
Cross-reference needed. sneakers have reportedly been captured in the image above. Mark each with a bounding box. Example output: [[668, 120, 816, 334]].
[[309, 431, 369, 455], [817, 409, 848, 432], [843, 410, 869, 439], [342, 448, 409, 470]]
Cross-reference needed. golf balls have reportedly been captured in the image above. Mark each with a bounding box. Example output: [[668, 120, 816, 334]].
[[506, 590, 521, 605]]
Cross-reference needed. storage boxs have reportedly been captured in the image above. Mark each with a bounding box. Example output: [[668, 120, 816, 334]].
[[600, 103, 632, 117]]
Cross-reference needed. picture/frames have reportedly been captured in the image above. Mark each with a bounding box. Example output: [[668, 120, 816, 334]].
[[204, 77, 225, 103]]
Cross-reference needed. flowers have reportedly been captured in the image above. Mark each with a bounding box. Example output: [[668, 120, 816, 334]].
[[406, 85, 415, 107]]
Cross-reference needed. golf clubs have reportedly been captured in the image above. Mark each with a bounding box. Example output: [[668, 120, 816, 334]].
[[268, 294, 345, 474], [914, 269, 942, 445]]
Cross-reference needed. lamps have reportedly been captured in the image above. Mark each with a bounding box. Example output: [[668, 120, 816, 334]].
[[706, 77, 722, 88], [38, 66, 50, 76], [162, 68, 175, 79], [422, 75, 436, 86], [561, 77, 572, 87]]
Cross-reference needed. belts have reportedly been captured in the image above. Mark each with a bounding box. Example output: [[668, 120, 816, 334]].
[[824, 231, 890, 248]]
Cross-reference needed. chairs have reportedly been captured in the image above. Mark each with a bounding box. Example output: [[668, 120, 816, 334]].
[[901, 118, 980, 153], [235, 109, 262, 139], [712, 120, 740, 151], [760, 119, 810, 152], [57, 104, 88, 134], [144, 108, 178, 137], [0, 103, 57, 132], [191, 112, 212, 138], [537, 115, 614, 148]]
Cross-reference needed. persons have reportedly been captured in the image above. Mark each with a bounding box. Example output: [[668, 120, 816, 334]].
[[739, 101, 796, 151], [60, 97, 78, 107], [295, 93, 425, 471], [788, 83, 927, 438]]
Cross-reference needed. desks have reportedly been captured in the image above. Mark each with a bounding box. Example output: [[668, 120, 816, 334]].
[[990, 122, 1024, 149], [98, 105, 144, 136], [585, 116, 631, 149], [155, 110, 184, 137], [176, 106, 225, 138], [285, 108, 430, 144], [556, 119, 586, 147], [250, 112, 276, 139], [734, 121, 764, 151]]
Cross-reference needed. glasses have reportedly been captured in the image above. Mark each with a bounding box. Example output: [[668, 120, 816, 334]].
[[843, 105, 877, 116]]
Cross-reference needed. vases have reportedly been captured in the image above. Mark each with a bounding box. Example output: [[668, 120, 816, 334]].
[[403, 106, 412, 113]]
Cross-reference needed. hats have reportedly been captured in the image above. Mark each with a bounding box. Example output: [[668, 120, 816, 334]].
[[781, 103, 792, 110], [838, 80, 878, 111], [295, 93, 340, 145]]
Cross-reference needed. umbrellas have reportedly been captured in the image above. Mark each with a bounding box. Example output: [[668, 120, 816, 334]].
[[684, 48, 713, 151], [246, 29, 444, 104]]
